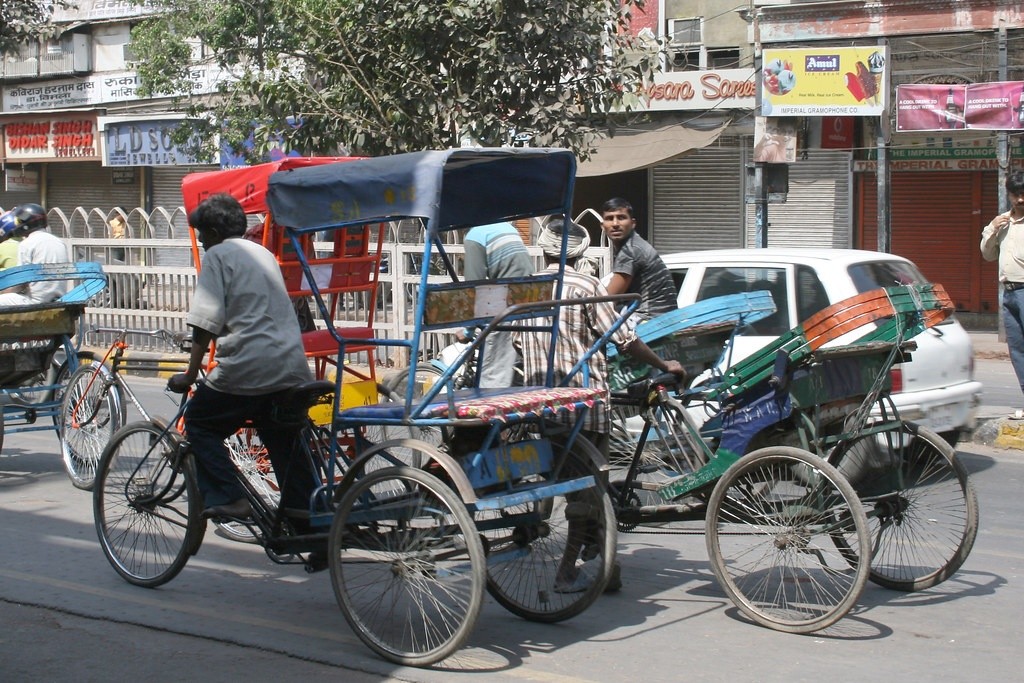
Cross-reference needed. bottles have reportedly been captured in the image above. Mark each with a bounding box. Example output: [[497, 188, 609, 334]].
[[1018, 91, 1024, 123], [945, 90, 958, 123]]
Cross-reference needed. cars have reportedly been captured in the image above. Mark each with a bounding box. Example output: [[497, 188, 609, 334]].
[[613, 246, 982, 486]]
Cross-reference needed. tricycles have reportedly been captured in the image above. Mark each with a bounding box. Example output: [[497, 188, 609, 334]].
[[88, 143, 629, 666], [60, 156, 425, 546], [0, 259, 127, 492], [596, 280, 983, 637]]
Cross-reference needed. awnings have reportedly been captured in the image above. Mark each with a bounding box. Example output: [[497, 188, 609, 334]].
[[523, 118, 734, 177]]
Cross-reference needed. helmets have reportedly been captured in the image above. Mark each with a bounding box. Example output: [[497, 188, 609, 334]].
[[12, 203, 48, 236], [0, 211, 16, 242]]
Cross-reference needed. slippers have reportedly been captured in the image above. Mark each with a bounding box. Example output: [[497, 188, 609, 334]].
[[555, 567, 594, 593], [304, 554, 328, 573], [604, 562, 623, 593], [1008, 410, 1024, 420], [199, 505, 255, 525]]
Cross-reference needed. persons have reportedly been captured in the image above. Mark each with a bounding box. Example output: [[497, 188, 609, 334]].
[[439, 222, 536, 391], [599, 196, 679, 322], [510, 214, 688, 592], [168, 194, 334, 574], [108, 207, 132, 261], [0, 204, 68, 305], [979, 170, 1024, 420]]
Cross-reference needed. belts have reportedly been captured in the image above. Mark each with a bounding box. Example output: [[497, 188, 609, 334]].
[[1003, 282, 1024, 291]]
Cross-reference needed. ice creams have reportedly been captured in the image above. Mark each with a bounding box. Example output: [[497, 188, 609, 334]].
[[867, 51, 885, 105], [763, 58, 796, 96]]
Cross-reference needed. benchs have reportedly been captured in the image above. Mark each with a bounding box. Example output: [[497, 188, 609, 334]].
[[276, 253, 382, 383], [696, 341, 918, 478], [0, 299, 89, 389], [328, 273, 644, 497]]
